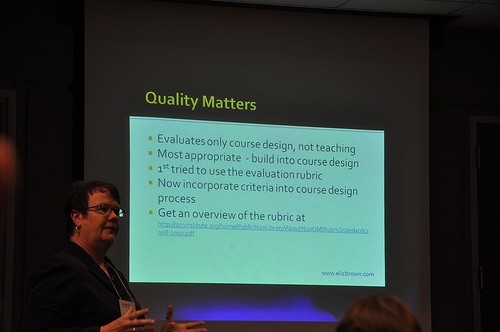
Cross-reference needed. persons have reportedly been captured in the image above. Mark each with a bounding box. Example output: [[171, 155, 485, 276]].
[[21, 179, 207, 332]]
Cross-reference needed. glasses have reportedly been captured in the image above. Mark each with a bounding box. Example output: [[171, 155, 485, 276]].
[[87, 203, 124, 219]]
